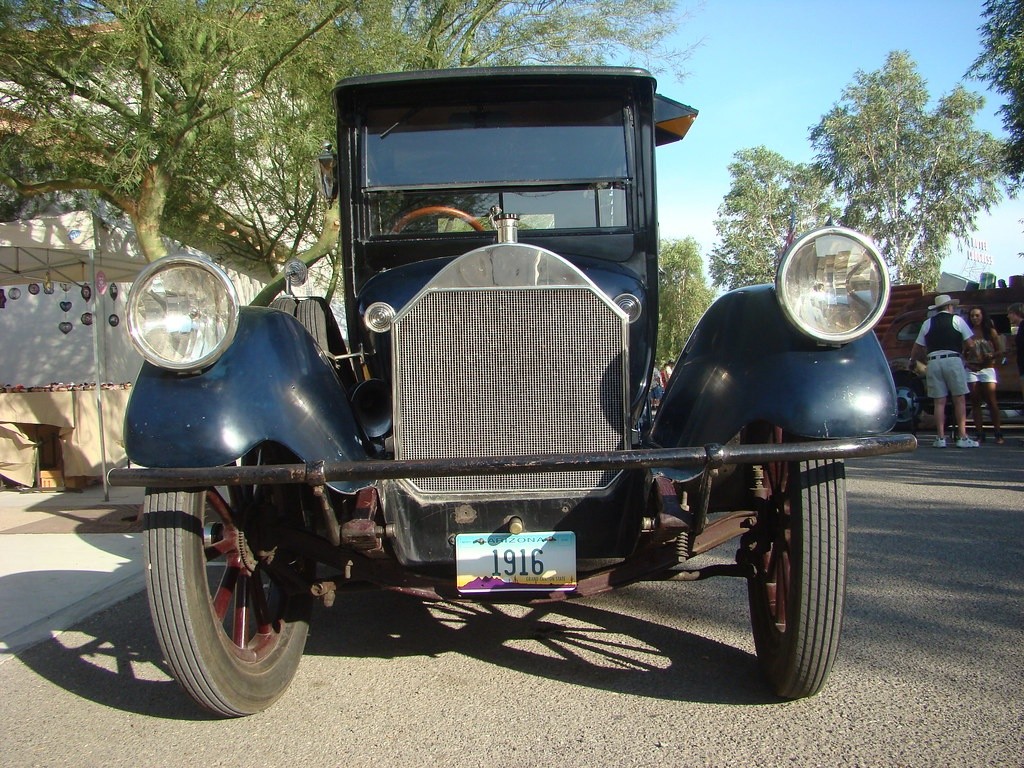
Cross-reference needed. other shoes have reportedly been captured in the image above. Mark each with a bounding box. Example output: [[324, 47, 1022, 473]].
[[995, 432, 1004, 444], [975, 434, 985, 444]]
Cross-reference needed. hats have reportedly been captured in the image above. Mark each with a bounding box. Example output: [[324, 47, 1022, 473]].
[[928, 295, 960, 310]]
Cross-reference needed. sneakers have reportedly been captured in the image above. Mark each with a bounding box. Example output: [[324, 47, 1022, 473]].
[[956, 435, 979, 448], [933, 437, 946, 447]]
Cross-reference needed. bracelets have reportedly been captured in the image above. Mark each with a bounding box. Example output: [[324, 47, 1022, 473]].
[[910, 358, 913, 361]]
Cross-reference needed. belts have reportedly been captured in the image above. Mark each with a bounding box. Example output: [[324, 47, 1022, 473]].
[[928, 354, 959, 361]]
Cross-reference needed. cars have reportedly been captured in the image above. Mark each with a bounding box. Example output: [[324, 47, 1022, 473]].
[[105, 62, 921, 719]]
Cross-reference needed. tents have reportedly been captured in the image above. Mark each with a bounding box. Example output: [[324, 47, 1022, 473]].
[[0, 208, 210, 504]]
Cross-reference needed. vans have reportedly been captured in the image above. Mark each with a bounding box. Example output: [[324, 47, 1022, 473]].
[[878, 299, 1024, 436]]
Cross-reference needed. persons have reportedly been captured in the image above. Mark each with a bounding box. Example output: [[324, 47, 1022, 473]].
[[963, 306, 1006, 444], [649, 358, 676, 409], [908, 294, 981, 447], [1007, 303, 1024, 376]]
